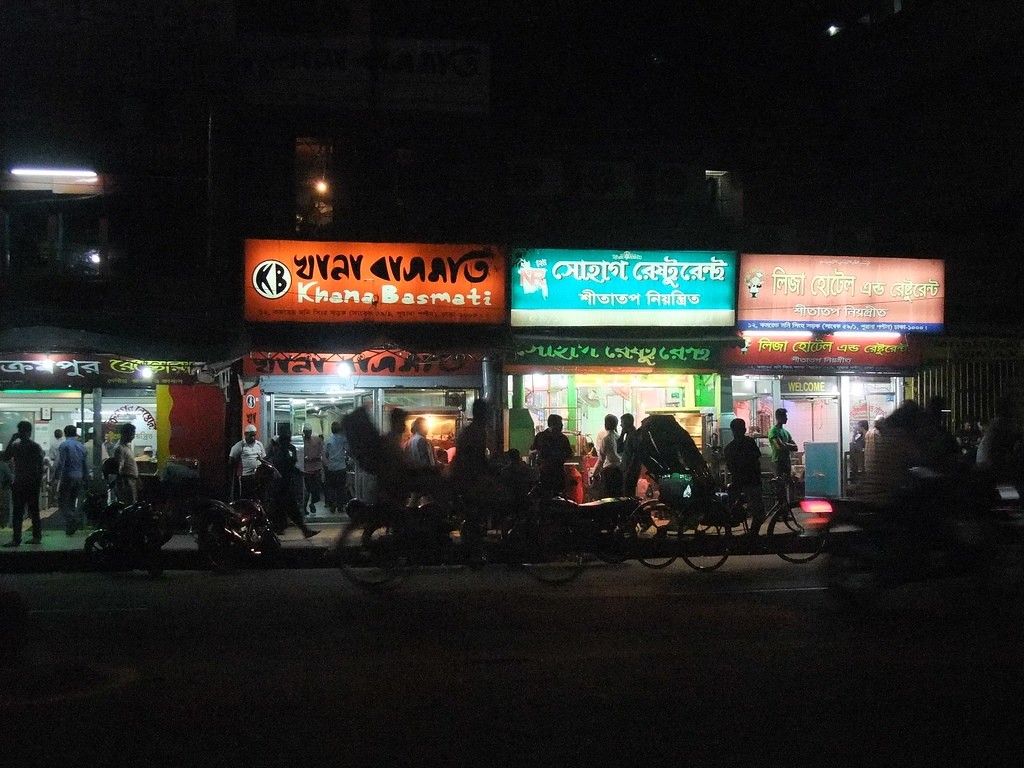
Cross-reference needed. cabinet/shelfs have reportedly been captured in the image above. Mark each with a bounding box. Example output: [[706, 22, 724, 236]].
[[645, 407, 703, 450]]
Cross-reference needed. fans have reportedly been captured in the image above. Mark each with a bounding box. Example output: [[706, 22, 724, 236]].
[[579, 389, 601, 419], [603, 386, 631, 408]]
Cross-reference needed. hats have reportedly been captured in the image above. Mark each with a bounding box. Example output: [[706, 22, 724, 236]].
[[303, 423, 312, 430], [245, 424, 257, 432]]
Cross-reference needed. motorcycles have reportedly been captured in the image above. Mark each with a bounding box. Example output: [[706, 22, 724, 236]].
[[504, 482, 652, 570], [83, 456, 280, 575]]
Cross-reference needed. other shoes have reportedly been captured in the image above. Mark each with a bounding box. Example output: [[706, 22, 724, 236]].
[[26, 538, 41, 545], [65, 523, 78, 535], [3, 540, 22, 547], [778, 515, 794, 522], [305, 530, 321, 539], [304, 508, 309, 514], [330, 508, 335, 513], [309, 502, 316, 513], [337, 507, 343, 512]]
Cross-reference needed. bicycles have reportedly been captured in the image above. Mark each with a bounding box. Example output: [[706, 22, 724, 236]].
[[335, 465, 525, 589], [624, 464, 831, 573]]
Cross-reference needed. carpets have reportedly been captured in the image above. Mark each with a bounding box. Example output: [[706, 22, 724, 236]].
[[25, 510, 67, 531]]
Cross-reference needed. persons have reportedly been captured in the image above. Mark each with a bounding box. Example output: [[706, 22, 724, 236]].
[[0, 422, 153, 548], [845, 400, 1024, 496], [767, 404, 798, 525], [227, 397, 762, 537]]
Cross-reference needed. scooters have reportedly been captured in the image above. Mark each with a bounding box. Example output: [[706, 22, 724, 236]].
[[827, 494, 1023, 622]]
